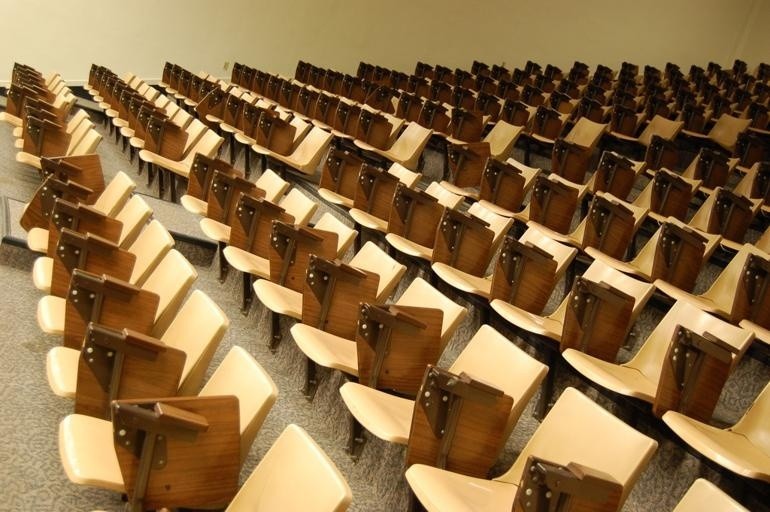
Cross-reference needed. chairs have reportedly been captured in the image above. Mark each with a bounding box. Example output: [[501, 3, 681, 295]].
[[1, 54, 768, 511]]
